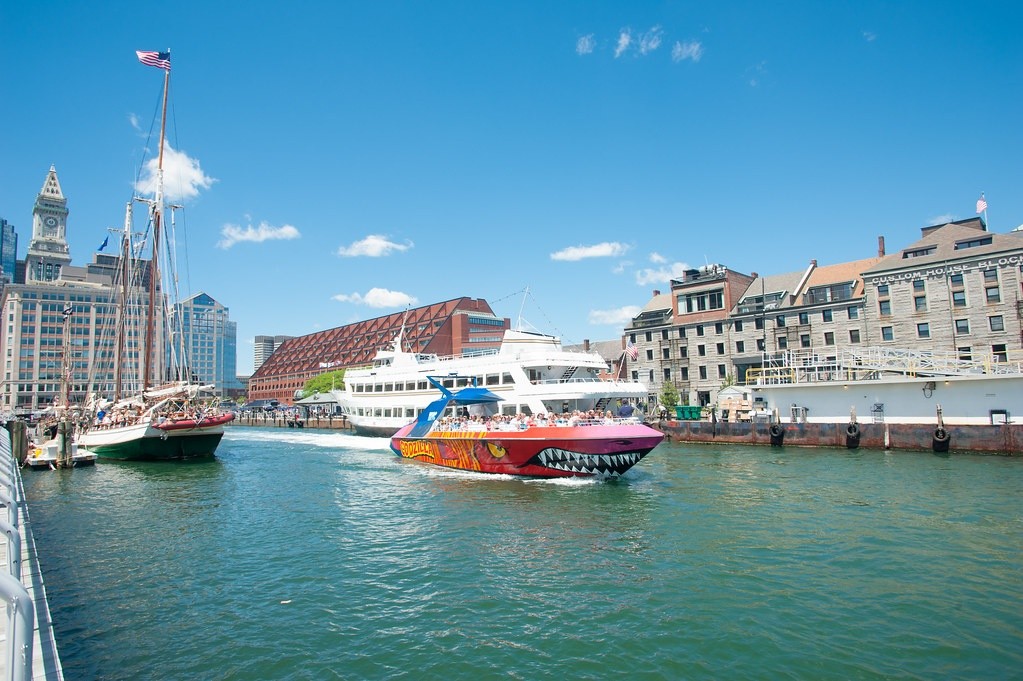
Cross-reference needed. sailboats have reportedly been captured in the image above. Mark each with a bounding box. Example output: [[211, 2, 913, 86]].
[[15, 48, 237, 470]]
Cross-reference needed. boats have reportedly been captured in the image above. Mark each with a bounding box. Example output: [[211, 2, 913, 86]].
[[328, 285, 651, 445], [386, 371, 663, 483]]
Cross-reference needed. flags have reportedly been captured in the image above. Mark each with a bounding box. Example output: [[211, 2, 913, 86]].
[[626, 340, 639, 361], [135, 49, 171, 72], [97, 237, 107, 251], [976, 196, 986, 215]]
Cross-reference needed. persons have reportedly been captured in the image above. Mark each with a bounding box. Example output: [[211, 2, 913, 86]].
[[64, 403, 220, 429], [615, 400, 634, 417], [434, 409, 615, 430], [228, 409, 332, 418]]
